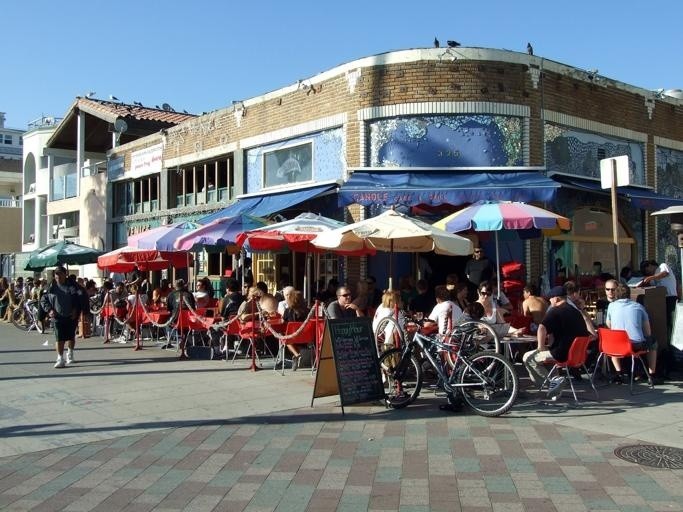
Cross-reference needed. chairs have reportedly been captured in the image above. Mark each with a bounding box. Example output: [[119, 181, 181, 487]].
[[592, 327, 652, 395], [538, 335, 601, 402], [102, 293, 443, 376]]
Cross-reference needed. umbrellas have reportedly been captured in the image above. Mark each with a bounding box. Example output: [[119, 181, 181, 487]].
[[22, 240, 106, 278], [431, 199, 570, 296], [309, 205, 474, 291], [235, 212, 376, 309], [97, 245, 192, 281], [127, 220, 226, 286], [172, 213, 288, 295]]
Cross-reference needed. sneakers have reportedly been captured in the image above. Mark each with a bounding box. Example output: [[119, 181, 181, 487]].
[[67, 353, 73, 363], [648, 377, 664, 385], [54, 358, 65, 368], [546, 376, 566, 398]]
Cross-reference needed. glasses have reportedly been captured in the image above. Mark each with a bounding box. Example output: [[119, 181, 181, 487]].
[[481, 292, 490, 296], [605, 288, 615, 291], [340, 293, 352, 297]]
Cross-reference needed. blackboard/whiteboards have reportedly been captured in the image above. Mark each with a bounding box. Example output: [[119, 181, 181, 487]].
[[328, 317, 385, 406]]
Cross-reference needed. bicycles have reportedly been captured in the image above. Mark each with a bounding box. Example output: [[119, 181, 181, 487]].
[[11, 281, 44, 334], [373, 316, 500, 398], [377, 304, 520, 417]]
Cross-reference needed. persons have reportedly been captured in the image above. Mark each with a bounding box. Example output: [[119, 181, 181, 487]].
[[0, 276, 52, 332], [522, 286, 589, 400], [67, 273, 387, 377], [522, 287, 550, 334], [408, 278, 514, 347], [373, 291, 408, 384], [562, 282, 599, 385], [588, 259, 680, 386], [465, 245, 496, 285], [43, 265, 87, 368]]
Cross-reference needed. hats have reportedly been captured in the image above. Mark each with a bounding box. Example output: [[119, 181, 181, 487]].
[[547, 286, 567, 298], [53, 266, 66, 275]]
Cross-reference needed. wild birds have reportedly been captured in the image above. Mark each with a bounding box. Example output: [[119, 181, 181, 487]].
[[446, 40, 461, 47], [100, 95, 144, 112], [434, 36, 439, 48], [527, 42, 533, 54], [86, 90, 96, 97], [183, 109, 187, 113], [155, 104, 160, 109]]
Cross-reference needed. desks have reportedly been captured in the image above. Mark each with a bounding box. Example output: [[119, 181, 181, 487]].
[[497, 332, 537, 391]]
